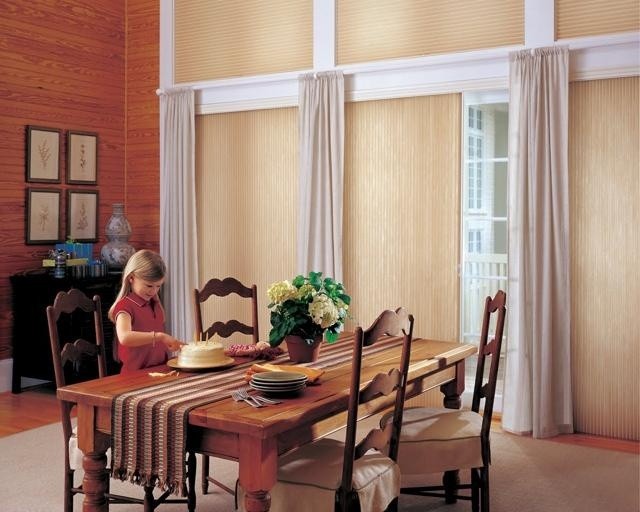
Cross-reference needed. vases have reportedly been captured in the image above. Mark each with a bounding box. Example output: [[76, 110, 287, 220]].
[[286, 335, 325, 361], [100, 204, 135, 270]]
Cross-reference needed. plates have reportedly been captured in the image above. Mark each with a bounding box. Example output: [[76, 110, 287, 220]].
[[167, 358, 234, 371], [248, 372, 309, 396]]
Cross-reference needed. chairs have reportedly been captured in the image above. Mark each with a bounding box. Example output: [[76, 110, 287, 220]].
[[195, 278, 268, 493], [235, 306, 414, 512], [380, 292, 506, 512], [46, 290, 198, 512]]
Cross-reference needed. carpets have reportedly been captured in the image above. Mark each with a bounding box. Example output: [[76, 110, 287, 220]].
[[0, 409, 640, 512]]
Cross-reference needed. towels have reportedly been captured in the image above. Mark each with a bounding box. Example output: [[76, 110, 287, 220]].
[[245, 362, 326, 388]]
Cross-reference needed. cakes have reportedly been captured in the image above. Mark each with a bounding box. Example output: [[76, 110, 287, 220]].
[[178, 341, 224, 365]]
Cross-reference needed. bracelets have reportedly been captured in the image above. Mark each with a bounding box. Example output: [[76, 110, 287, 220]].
[[152, 331, 155, 349]]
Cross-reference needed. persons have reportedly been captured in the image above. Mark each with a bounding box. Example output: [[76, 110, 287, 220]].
[[108, 250, 188, 374]]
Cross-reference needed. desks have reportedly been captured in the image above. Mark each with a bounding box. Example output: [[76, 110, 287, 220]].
[[8, 270, 119, 393]]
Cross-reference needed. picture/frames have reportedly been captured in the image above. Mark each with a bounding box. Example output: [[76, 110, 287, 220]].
[[25, 124, 61, 184], [65, 188, 100, 244], [25, 188, 60, 246], [67, 129, 99, 185]]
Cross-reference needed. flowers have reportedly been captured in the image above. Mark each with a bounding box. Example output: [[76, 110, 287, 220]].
[[266, 272, 349, 347]]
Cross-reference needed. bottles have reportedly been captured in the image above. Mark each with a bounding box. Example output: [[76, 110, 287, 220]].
[[55, 249, 66, 278]]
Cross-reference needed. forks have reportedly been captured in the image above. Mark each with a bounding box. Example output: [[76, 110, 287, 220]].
[[231, 388, 264, 408]]
